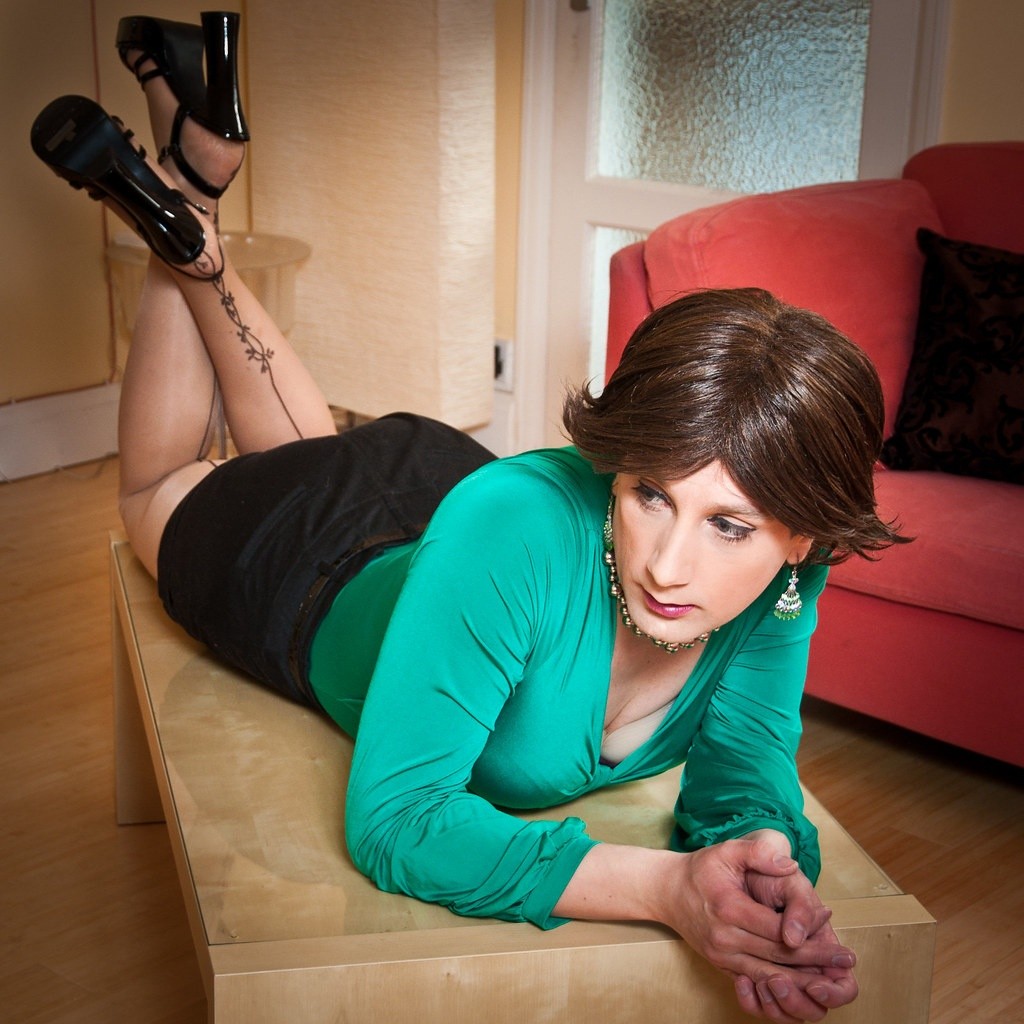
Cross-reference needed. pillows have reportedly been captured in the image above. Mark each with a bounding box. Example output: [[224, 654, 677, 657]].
[[882, 226, 1024, 485]]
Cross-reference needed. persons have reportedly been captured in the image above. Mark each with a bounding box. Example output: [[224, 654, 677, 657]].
[[29, 10, 918, 1024]]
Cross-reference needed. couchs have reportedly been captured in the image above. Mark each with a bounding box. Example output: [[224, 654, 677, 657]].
[[603, 142, 1024, 777]]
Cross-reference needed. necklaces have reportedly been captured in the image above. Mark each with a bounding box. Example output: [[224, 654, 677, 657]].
[[604, 496, 719, 652]]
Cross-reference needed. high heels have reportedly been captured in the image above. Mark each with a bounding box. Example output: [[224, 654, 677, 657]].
[[115, 10, 251, 199], [30, 94, 226, 281]]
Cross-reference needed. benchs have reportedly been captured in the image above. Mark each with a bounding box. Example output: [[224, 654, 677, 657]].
[[102, 526, 940, 1024]]
